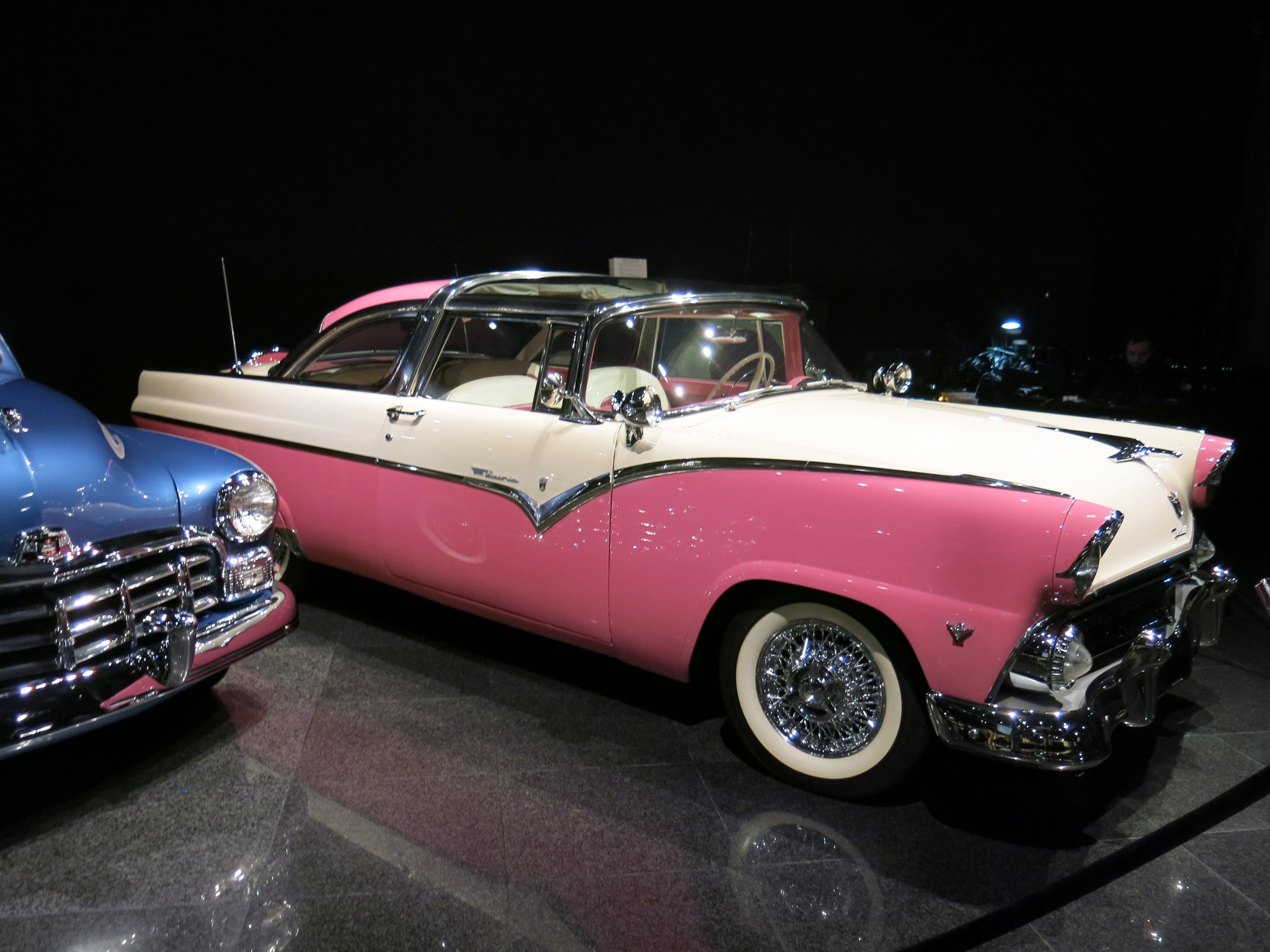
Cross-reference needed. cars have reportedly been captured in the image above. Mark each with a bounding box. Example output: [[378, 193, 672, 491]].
[[128, 254, 1242, 806], [1, 327, 305, 784]]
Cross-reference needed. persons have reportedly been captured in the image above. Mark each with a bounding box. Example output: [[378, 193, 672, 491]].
[[1094, 331, 1171, 417]]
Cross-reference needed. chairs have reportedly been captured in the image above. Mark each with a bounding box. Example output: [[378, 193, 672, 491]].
[[584, 367, 669, 412], [440, 375, 537, 412]]
